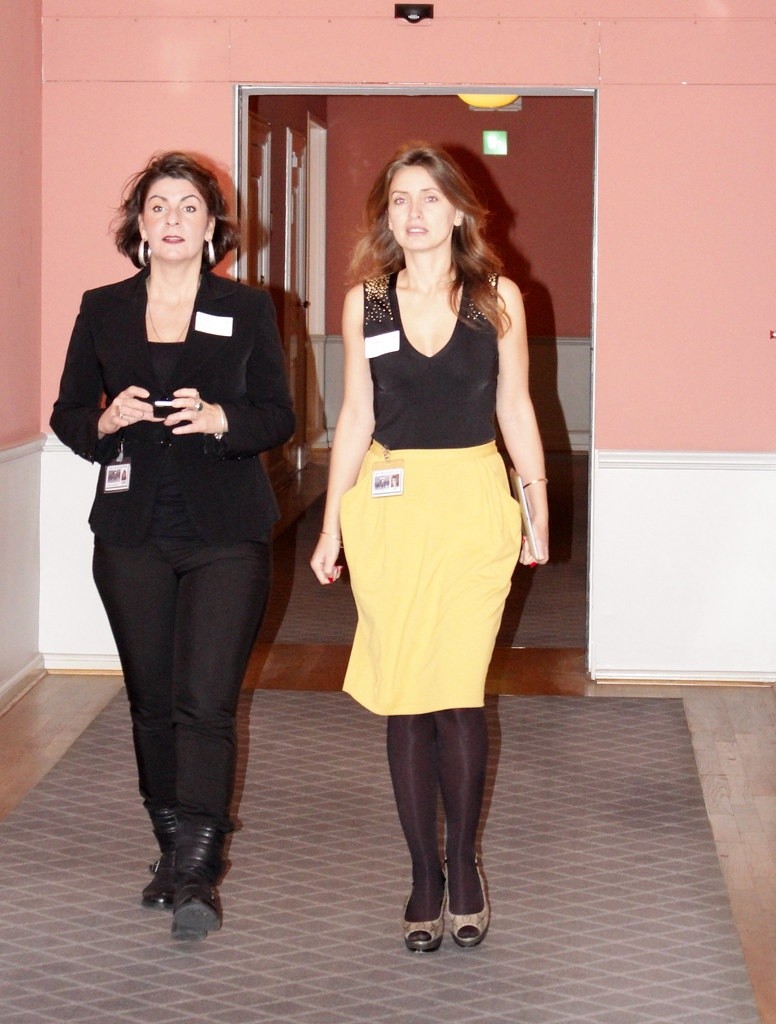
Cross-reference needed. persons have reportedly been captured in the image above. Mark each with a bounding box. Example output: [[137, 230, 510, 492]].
[[49, 148, 296, 941], [307, 142, 550, 956]]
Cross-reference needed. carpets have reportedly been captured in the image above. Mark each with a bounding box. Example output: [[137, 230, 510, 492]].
[[253, 452, 587, 649], [0, 683, 760, 1024]]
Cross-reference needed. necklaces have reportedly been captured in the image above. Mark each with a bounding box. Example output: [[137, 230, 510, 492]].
[[147, 303, 190, 342]]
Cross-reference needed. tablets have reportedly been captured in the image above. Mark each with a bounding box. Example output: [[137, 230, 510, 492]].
[[509, 468, 539, 561]]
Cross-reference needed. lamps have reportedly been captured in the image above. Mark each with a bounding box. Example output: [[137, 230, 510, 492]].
[[458, 94, 519, 109], [395, 3, 434, 24]]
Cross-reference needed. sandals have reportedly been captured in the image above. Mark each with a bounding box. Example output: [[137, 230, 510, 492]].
[[401, 865, 446, 951], [444, 851, 490, 946]]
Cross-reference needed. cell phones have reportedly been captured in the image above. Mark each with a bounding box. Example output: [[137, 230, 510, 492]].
[[153, 401, 184, 418]]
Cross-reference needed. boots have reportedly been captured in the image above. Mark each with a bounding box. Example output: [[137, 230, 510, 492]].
[[171, 820, 223, 941], [141, 809, 178, 910]]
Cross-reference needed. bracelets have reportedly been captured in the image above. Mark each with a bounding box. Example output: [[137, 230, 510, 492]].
[[320, 531, 342, 542], [523, 478, 548, 489]]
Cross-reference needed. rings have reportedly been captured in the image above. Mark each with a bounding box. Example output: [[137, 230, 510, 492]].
[[117, 412, 122, 419], [115, 405, 122, 410], [194, 401, 203, 412]]
